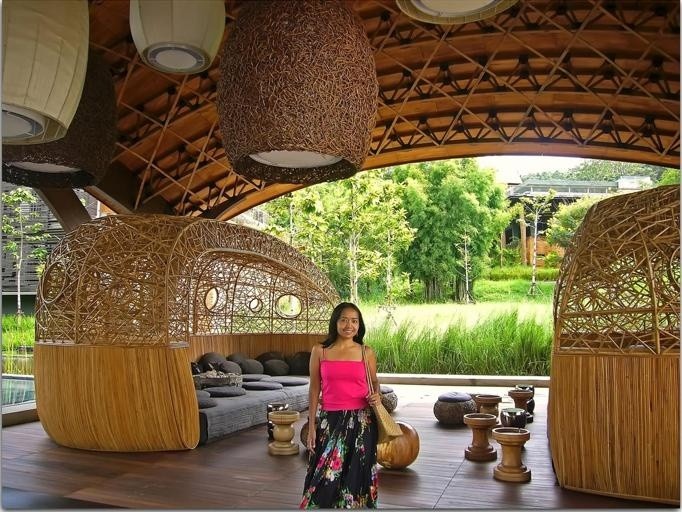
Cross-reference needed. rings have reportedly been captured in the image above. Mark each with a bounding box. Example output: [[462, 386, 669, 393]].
[[374, 400, 378, 403]]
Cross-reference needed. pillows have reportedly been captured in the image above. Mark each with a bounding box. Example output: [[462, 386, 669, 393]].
[[191, 351, 309, 408]]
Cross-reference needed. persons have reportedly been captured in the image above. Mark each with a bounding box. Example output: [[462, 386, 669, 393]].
[[300, 302, 381, 509]]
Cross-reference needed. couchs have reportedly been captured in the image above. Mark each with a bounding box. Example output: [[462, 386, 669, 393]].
[[189, 350, 310, 447]]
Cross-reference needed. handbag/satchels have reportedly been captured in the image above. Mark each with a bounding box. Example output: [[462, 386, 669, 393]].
[[361, 344, 404, 445]]
